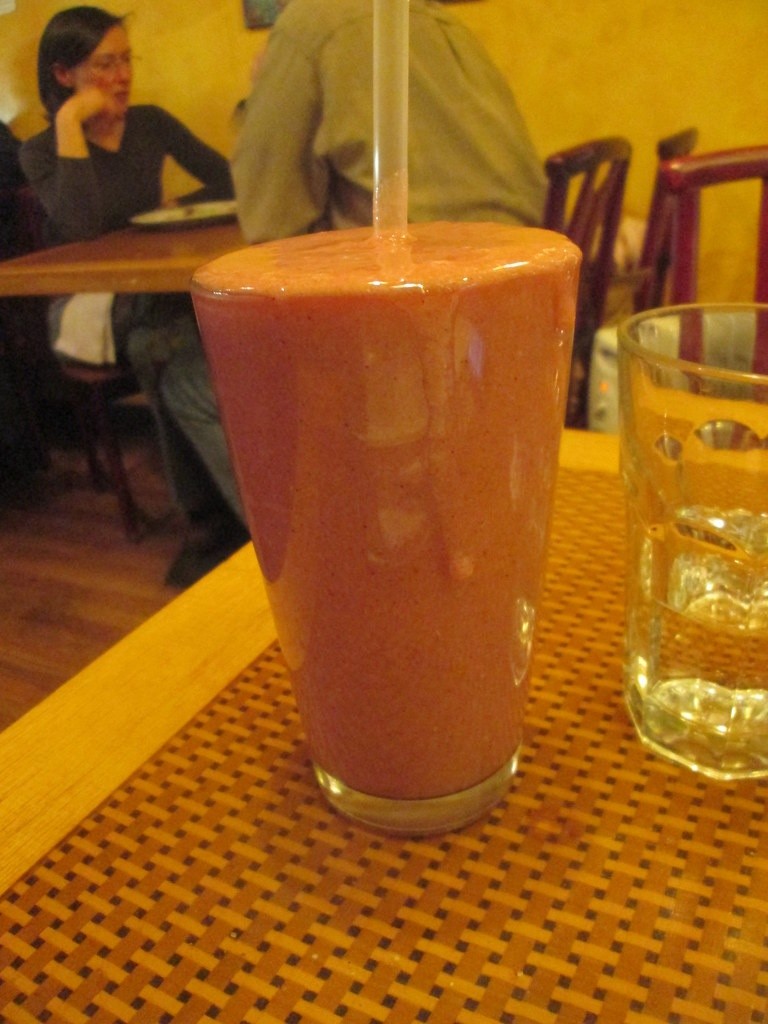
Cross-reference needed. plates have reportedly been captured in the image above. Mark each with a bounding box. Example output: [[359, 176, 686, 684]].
[[130, 201, 236, 225]]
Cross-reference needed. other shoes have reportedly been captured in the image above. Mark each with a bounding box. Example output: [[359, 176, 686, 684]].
[[183, 501, 229, 548]]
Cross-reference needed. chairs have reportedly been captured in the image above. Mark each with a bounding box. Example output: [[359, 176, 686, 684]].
[[636, 129, 696, 312], [655, 146, 767, 370], [543, 138, 633, 430], [13, 184, 149, 546]]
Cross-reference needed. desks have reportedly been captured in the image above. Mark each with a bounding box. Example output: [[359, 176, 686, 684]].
[[1, 222, 247, 592], [2, 431, 768, 1024]]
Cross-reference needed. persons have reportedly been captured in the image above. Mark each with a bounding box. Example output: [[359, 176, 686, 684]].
[[18, 5, 234, 369], [159, 0, 551, 539]]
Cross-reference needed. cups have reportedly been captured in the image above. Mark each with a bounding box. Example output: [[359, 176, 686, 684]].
[[617, 301, 768, 779], [191, 221, 583, 838]]
